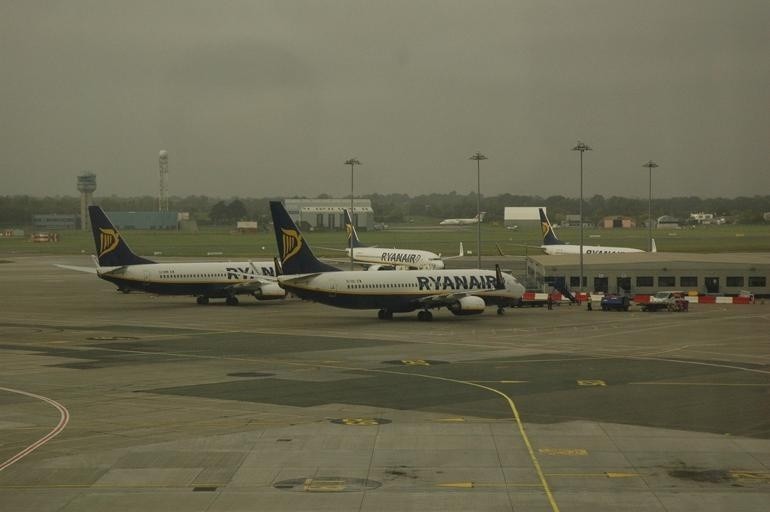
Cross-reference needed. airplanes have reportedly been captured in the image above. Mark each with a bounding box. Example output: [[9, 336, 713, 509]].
[[230, 198, 526, 319], [504, 206, 661, 254], [50, 203, 295, 306]]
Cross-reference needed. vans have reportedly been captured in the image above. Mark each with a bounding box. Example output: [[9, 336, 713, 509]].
[[648, 290, 689, 312]]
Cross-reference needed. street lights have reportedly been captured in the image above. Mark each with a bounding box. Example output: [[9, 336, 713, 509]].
[[643, 158, 659, 253], [570, 137, 591, 291], [467, 150, 489, 268], [345, 156, 363, 270]]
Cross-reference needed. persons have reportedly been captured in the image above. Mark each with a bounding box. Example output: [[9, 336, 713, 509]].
[[603, 292, 610, 311], [587, 294, 592, 311], [667, 294, 685, 312], [548, 293, 553, 311]]
